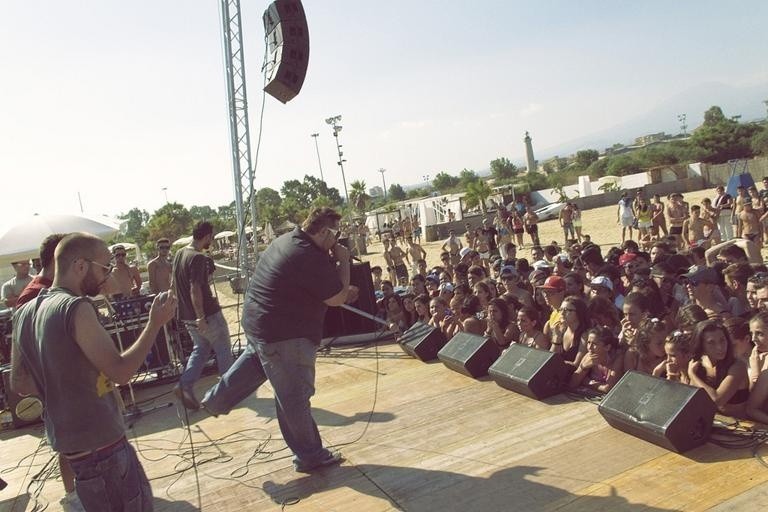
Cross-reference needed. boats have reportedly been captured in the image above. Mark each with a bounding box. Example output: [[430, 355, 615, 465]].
[[535, 201, 568, 223]]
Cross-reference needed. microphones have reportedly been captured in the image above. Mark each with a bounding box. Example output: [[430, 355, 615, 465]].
[[160, 292, 176, 337], [331, 245, 360, 262]]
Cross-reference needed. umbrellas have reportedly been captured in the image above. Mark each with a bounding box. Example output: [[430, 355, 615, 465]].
[[107, 242, 135, 253], [173, 237, 191, 245], [0, 212, 120, 264], [214, 230, 236, 239], [245, 226, 262, 233]]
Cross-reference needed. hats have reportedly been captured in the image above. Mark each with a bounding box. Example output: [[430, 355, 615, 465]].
[[483, 218, 490, 225], [743, 197, 752, 206], [380, 242, 721, 290]]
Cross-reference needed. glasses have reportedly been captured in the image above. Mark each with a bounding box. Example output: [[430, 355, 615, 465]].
[[74, 258, 113, 274], [327, 228, 341, 240], [14, 263, 31, 266], [115, 253, 126, 257], [558, 308, 577, 313], [158, 246, 170, 249]]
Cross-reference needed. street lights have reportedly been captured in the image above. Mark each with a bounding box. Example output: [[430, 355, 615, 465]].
[[161, 187, 169, 202], [312, 132, 324, 184], [678, 113, 687, 137], [326, 114, 361, 261], [378, 167, 387, 198]]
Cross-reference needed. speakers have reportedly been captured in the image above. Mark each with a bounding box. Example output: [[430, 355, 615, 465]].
[[437, 331, 498, 378], [397, 321, 444, 362], [262, 0, 309, 104], [488, 343, 569, 399], [598, 368, 717, 454]]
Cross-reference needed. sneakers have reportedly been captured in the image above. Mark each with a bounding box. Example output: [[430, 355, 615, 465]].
[[60, 490, 80, 504], [174, 386, 199, 410], [201, 402, 222, 418], [294, 452, 342, 472]]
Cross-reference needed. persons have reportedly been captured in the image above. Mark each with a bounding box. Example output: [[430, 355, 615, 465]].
[[9, 231, 180, 511], [199, 206, 359, 474], [442, 203, 585, 265], [360, 216, 421, 246], [15, 233, 79, 506], [616, 176, 768, 253], [0, 258, 35, 308], [146, 237, 173, 295], [370, 233, 767, 427], [108, 244, 142, 298], [32, 258, 42, 275], [172, 219, 233, 411]]
